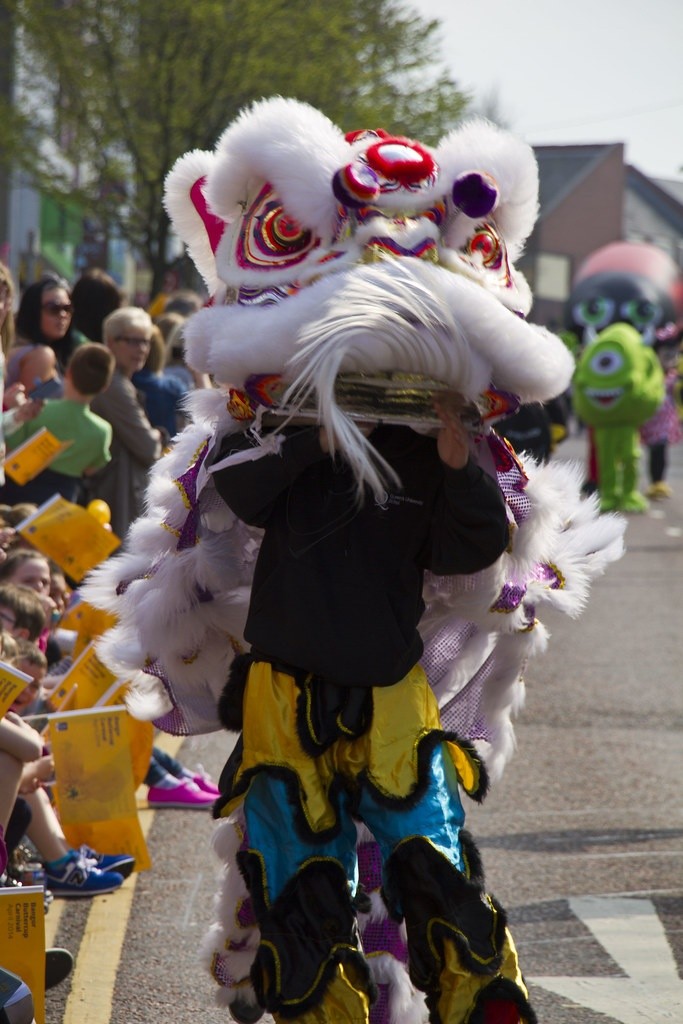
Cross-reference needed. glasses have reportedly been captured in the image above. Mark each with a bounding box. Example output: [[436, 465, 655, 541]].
[[41, 301, 73, 315], [115, 334, 152, 347]]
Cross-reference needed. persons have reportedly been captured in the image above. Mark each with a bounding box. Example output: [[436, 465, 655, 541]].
[[638, 340, 683, 497], [0, 255, 221, 1024]]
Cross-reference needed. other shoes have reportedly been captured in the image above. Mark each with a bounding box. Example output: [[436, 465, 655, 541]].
[[45, 949, 74, 989], [646, 482, 669, 500]]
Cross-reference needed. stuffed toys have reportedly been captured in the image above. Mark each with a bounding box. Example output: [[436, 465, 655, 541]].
[[78, 95, 625, 1024]]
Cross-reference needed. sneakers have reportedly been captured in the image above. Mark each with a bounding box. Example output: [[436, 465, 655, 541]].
[[147, 762, 221, 808], [7, 860, 54, 914], [43, 845, 136, 898]]
